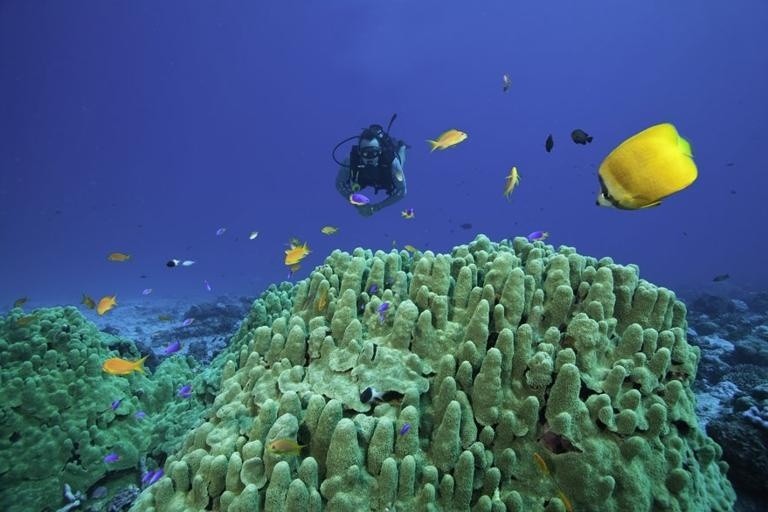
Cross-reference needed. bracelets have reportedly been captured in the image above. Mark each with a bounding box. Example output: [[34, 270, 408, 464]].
[[368, 204, 375, 216]]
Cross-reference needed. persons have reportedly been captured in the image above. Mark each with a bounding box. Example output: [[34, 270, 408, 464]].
[[332, 126, 415, 218]]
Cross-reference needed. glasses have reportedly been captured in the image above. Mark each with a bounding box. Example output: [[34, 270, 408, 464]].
[[358, 149, 379, 159]]
